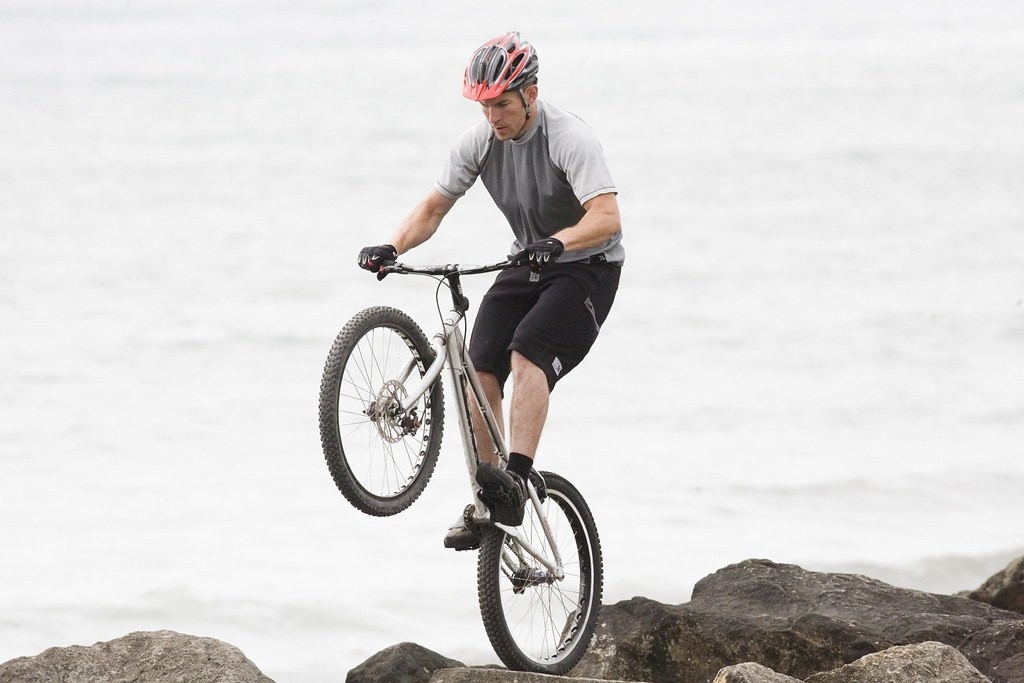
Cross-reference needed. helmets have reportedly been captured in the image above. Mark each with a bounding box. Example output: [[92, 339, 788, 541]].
[[462, 31, 539, 100]]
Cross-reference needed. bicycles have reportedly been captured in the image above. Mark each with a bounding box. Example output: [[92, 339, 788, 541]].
[[318, 259, 604, 675]]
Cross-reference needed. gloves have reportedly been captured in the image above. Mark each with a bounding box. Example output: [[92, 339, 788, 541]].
[[508, 238, 565, 273], [357, 244, 398, 281]]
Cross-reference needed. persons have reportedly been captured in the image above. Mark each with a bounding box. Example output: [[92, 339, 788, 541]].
[[357, 32, 626, 548]]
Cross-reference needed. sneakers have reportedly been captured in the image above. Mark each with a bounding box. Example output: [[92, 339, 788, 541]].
[[476, 463, 525, 527], [444, 525, 478, 548]]
